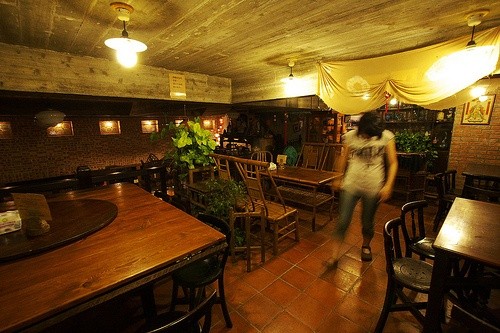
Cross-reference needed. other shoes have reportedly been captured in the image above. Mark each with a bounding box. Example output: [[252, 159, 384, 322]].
[[361, 246, 372, 262], [322, 257, 338, 269]]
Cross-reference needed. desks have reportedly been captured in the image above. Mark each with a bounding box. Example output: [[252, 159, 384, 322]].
[[462, 165, 500, 204], [422, 197, 500, 333], [257, 166, 343, 233], [1, 182, 227, 333], [189, 182, 218, 216]]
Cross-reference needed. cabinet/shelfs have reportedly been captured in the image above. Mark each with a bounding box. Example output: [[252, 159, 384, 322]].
[[309, 100, 455, 203]]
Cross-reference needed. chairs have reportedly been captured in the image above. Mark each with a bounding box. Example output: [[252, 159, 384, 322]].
[[374, 169, 500, 333], [0, 143, 343, 333]]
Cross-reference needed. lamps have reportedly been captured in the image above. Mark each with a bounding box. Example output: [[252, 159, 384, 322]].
[[47, 121, 74, 138], [100, 120, 121, 136], [462, 9, 489, 48], [203, 119, 216, 129], [140, 120, 158, 134], [0, 121, 11, 139], [35, 98, 66, 127], [104, 2, 147, 68]]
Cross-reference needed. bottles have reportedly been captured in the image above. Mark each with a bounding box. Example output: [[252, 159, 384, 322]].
[[278, 159, 284, 170], [379, 101, 454, 151]]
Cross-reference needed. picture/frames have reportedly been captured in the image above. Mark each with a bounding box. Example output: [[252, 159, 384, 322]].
[[461, 94, 496, 125]]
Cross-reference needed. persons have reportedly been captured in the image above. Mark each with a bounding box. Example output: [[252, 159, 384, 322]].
[[322, 111, 399, 268]]
